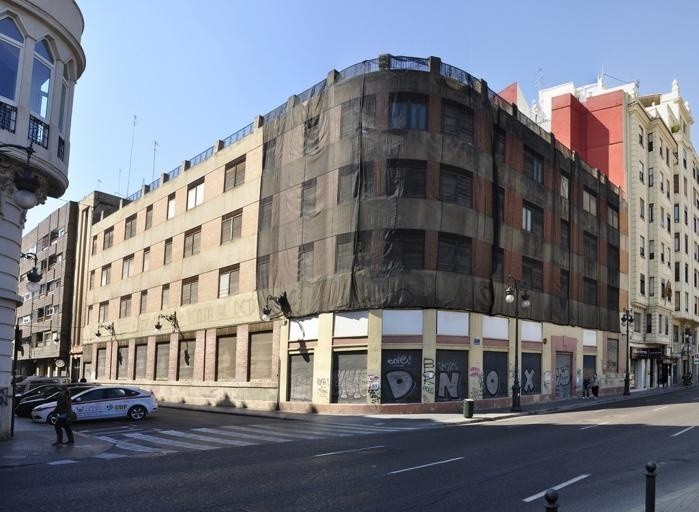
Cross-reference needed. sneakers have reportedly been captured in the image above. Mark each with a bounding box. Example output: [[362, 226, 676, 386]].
[[51, 439, 75, 447]]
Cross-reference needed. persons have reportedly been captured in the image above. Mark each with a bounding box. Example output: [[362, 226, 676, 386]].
[[582, 375, 591, 400], [590, 372, 600, 400], [50, 384, 76, 447], [79, 376, 87, 382]]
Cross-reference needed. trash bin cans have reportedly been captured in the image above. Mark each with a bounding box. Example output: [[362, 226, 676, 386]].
[[684, 375, 689, 386], [463, 399, 475, 418]]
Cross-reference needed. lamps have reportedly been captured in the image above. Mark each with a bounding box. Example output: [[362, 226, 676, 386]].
[[18, 250, 43, 294], [92, 320, 116, 340], [262, 293, 286, 323], [155, 310, 178, 336]]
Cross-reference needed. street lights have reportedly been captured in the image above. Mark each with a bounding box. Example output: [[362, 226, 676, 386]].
[[622, 307, 634, 395], [503, 273, 531, 413], [683, 325, 693, 375]]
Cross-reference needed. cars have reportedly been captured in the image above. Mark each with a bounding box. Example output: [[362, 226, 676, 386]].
[[11, 375, 159, 425]]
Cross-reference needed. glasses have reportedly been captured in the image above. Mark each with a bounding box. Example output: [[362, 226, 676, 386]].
[[58, 389, 64, 392]]
